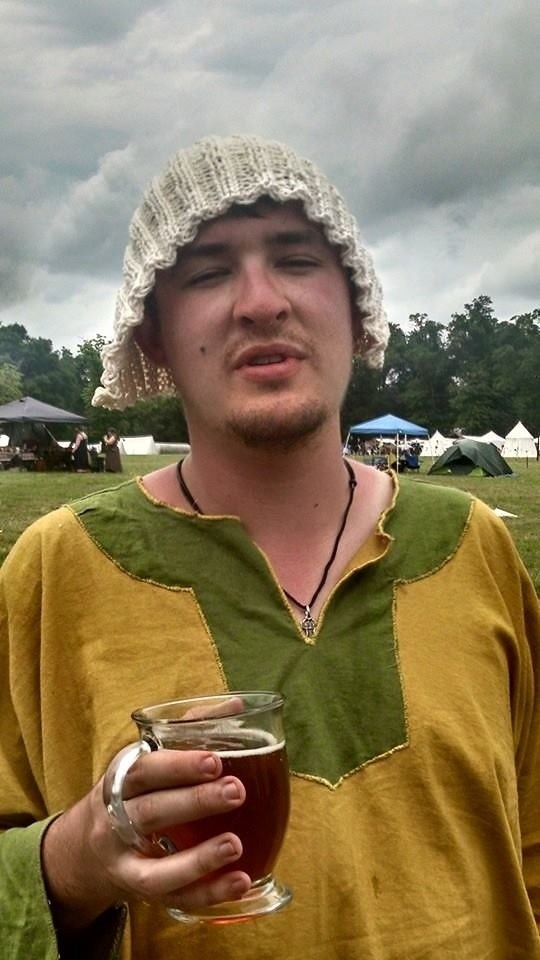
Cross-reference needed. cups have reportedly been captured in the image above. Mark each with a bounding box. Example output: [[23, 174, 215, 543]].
[[101, 690, 293, 927]]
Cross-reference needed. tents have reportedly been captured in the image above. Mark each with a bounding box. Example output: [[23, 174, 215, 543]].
[[0, 396, 89, 471], [343, 414, 435, 476], [420, 421, 538, 477]]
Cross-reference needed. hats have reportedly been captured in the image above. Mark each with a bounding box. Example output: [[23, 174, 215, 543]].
[[107, 427, 118, 432], [92, 133, 390, 412]]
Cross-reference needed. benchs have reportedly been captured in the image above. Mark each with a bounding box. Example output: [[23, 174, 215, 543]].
[[0, 446, 76, 473]]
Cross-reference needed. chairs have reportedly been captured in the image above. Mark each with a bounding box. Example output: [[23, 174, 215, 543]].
[[370, 448, 421, 473]]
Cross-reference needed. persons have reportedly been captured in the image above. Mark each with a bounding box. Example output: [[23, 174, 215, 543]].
[[104, 427, 123, 474], [72, 424, 88, 472], [0, 134, 539, 960], [348, 435, 423, 463]]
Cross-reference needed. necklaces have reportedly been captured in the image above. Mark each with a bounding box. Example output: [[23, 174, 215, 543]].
[[178, 458, 358, 637]]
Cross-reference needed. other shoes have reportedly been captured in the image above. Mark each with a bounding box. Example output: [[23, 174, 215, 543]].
[[76, 469, 88, 473]]
